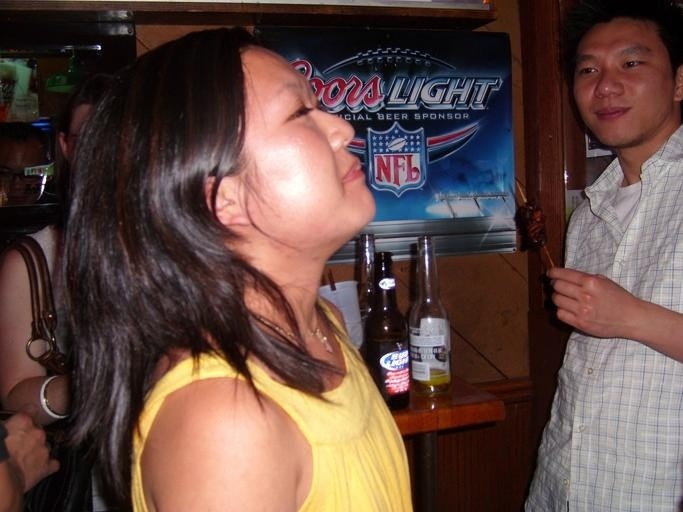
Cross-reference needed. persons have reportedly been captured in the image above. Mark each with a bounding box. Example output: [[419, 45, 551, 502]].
[[55, 29, 414, 512], [520, 2, 683, 511]]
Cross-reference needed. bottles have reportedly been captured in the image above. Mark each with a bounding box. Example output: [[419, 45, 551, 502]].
[[362, 249, 411, 410], [348, 233, 375, 311], [405, 234, 453, 401]]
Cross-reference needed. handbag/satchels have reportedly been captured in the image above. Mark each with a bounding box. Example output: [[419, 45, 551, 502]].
[[1, 354, 99, 512]]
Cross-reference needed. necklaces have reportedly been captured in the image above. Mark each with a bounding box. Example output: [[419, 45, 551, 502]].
[[256, 306, 334, 354]]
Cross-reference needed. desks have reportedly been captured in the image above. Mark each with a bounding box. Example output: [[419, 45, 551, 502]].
[[389, 370, 506, 512]]
